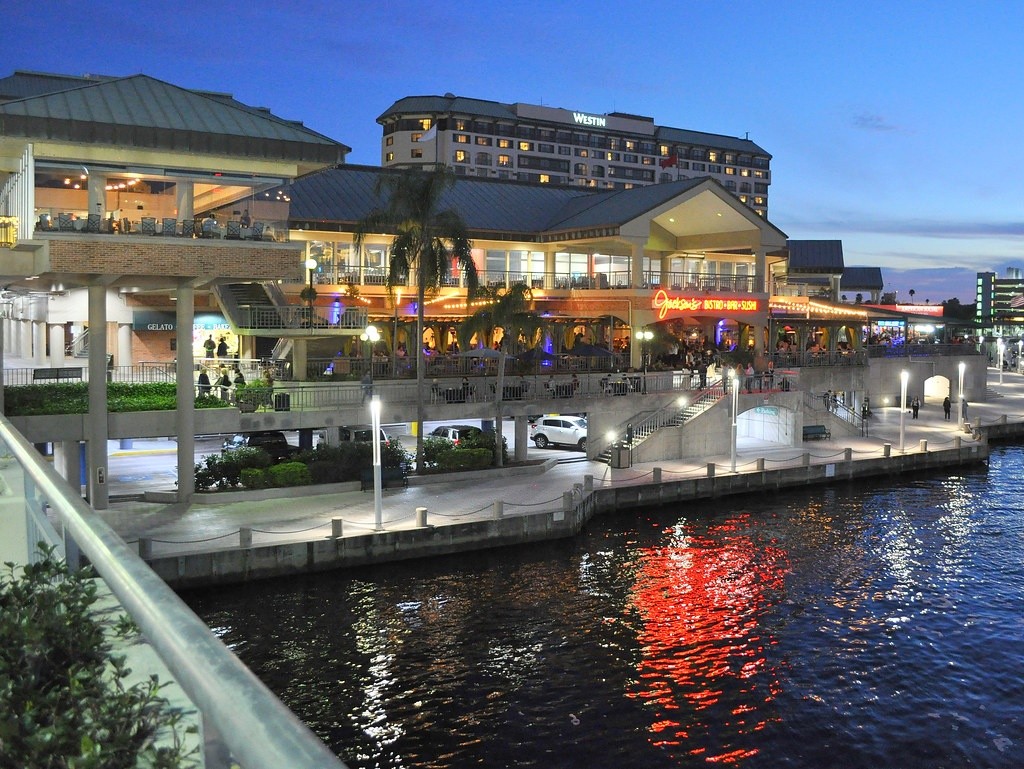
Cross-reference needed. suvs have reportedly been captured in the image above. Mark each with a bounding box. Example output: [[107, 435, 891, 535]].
[[317, 426, 388, 452], [425, 425, 481, 447], [530, 416, 588, 452]]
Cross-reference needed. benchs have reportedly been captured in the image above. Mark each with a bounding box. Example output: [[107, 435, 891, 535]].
[[896, 396, 912, 408], [34, 367, 82, 384], [803, 425, 831, 442], [360, 468, 409, 494]]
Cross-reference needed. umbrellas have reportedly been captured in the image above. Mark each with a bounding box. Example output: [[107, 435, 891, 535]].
[[560, 344, 613, 390], [454, 348, 514, 402], [510, 347, 560, 393]]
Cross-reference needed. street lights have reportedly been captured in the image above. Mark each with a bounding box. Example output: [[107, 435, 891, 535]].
[[1018, 340, 1021, 373], [372, 395, 381, 530], [636, 331, 655, 393], [360, 325, 380, 394], [306, 259, 317, 327], [730, 379, 739, 472], [996, 338, 1004, 384], [900, 369, 907, 452], [958, 360, 964, 430]]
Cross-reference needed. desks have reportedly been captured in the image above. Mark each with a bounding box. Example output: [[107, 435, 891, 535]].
[[138, 224, 190, 228], [53, 220, 109, 230], [212, 227, 252, 232]]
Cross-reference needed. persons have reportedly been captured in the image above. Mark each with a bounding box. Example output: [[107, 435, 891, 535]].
[[911, 396, 920, 419], [107, 355, 114, 381], [943, 397, 952, 419], [263, 370, 273, 408], [203, 334, 228, 366], [962, 399, 968, 419], [350, 325, 978, 406], [239, 209, 251, 229], [208, 213, 217, 228], [823, 390, 838, 412], [199, 368, 244, 394]]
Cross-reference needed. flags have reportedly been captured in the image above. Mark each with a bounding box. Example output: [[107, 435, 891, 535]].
[[1011, 293, 1024, 307]]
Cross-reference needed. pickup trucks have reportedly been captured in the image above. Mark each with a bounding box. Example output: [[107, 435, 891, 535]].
[[221, 432, 302, 466]]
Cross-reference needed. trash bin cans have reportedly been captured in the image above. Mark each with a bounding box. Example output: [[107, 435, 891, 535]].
[[865, 397, 869, 414], [275, 393, 290, 411], [611, 441, 630, 469], [629, 376, 641, 392]]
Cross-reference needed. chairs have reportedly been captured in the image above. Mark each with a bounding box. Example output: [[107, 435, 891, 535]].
[[244, 222, 264, 240], [142, 217, 158, 235], [162, 218, 176, 236], [179, 220, 194, 238], [600, 378, 638, 396], [40, 212, 131, 232], [490, 381, 530, 401], [430, 381, 477, 405], [194, 220, 212, 238], [344, 271, 738, 290], [224, 221, 240, 240], [544, 380, 581, 399]]
